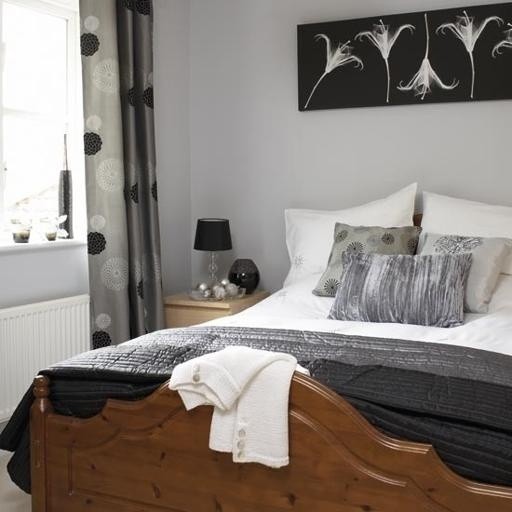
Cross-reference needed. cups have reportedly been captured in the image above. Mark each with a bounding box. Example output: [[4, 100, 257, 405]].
[[40, 216, 60, 241], [11, 219, 32, 243], [190, 278, 246, 302]]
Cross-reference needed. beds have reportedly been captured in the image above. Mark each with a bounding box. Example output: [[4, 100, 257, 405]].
[[0, 210, 512, 510]]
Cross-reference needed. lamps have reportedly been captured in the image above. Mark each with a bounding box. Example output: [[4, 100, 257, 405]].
[[188, 216, 238, 301]]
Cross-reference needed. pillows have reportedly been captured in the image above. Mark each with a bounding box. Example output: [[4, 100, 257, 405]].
[[283, 181, 512, 328]]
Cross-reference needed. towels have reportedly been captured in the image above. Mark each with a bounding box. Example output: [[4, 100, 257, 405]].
[[166, 343, 314, 473]]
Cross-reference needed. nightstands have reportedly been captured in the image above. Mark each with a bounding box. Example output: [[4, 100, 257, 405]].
[[161, 288, 271, 329]]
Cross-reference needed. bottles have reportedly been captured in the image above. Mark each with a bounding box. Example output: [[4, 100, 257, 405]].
[[228, 258, 260, 294]]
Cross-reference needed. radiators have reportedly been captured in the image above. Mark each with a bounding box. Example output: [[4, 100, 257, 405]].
[[0, 293, 94, 424]]
